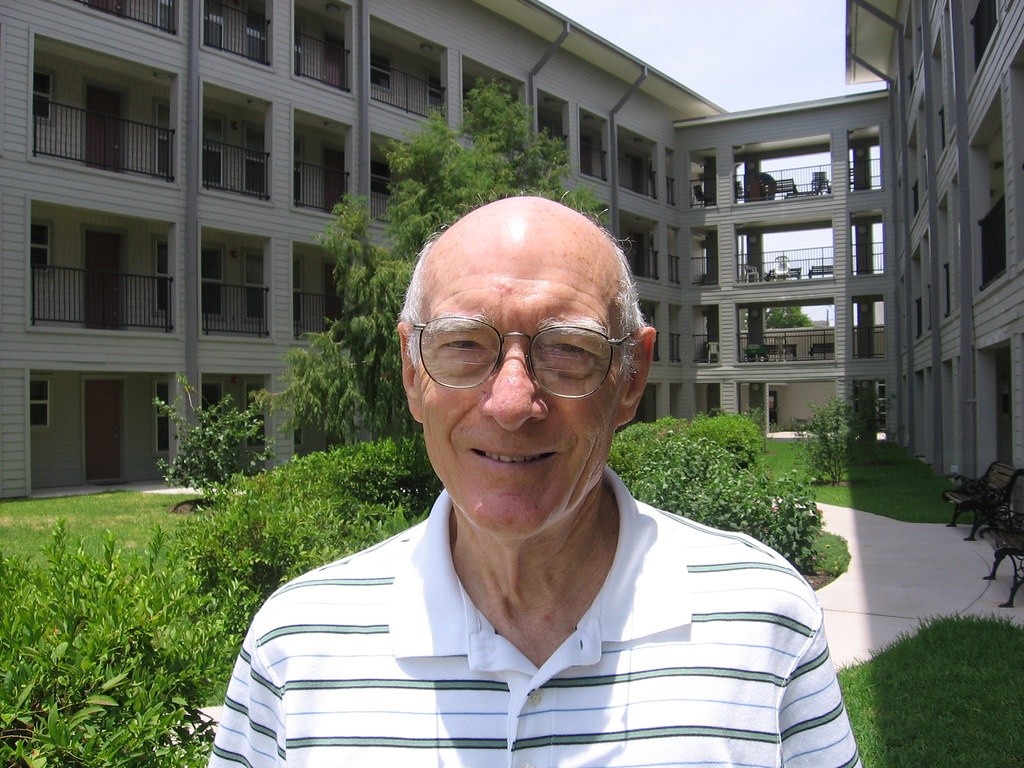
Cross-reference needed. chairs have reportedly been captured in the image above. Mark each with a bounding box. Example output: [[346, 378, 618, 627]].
[[745, 265, 759, 283], [706, 342, 719, 363], [694, 185, 711, 207]]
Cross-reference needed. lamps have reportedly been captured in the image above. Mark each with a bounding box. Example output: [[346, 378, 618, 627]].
[[327, 4, 340, 12], [421, 44, 433, 52]]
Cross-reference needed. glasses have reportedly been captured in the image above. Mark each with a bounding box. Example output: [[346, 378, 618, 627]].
[[412, 316, 643, 399]]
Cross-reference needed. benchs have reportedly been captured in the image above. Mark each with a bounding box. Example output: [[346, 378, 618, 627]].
[[758, 179, 818, 199], [764, 344, 797, 360], [767, 268, 801, 280], [809, 343, 835, 359], [808, 266, 833, 279], [943, 461, 1024, 608]]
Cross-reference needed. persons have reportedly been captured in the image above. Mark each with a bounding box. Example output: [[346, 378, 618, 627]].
[[209, 193, 858, 768]]
